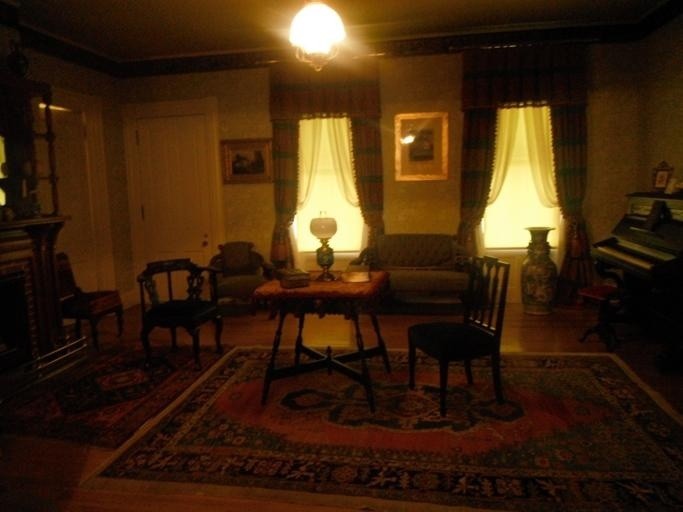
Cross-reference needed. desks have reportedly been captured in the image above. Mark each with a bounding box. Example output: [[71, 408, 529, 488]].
[[252, 269, 392, 413]]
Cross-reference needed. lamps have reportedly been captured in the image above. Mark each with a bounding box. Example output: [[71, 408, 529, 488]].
[[310, 212, 337, 282], [287, 0, 346, 74]]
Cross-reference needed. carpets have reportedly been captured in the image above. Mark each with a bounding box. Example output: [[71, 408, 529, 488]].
[[78, 343, 682, 512], [1, 343, 234, 451]]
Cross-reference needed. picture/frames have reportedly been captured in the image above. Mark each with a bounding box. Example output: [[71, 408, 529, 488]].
[[219, 137, 275, 185], [393, 112, 450, 182], [651, 159, 674, 192]]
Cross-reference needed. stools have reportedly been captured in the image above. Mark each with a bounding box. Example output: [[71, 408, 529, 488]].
[[577, 284, 626, 353]]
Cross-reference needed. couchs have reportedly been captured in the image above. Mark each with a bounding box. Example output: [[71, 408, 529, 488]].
[[350, 233, 483, 311]]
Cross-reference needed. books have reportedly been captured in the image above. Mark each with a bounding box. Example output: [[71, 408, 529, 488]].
[[644, 200, 666, 232]]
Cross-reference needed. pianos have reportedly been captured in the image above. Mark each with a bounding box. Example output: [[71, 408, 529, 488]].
[[591, 192, 683, 332]]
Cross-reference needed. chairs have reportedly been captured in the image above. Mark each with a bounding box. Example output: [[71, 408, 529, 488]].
[[137, 258, 225, 371], [209, 241, 265, 317], [407, 255, 510, 417], [53, 250, 124, 348]]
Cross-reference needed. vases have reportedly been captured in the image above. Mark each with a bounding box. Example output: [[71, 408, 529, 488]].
[[520, 226, 558, 317]]
